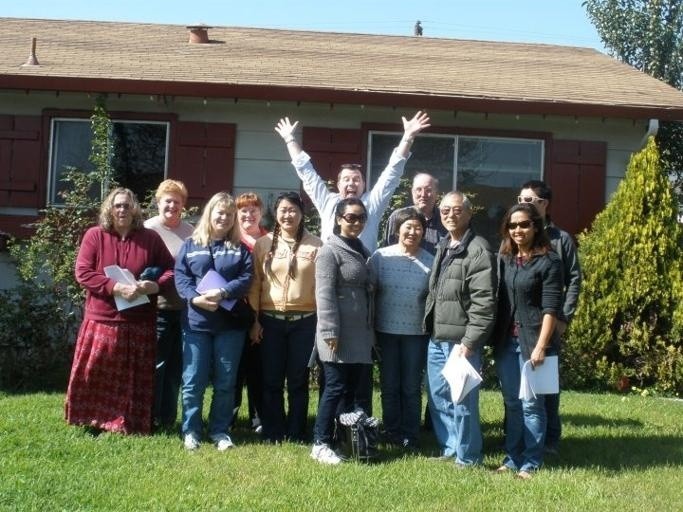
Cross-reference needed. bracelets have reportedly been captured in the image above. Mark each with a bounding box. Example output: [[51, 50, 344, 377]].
[[285, 139, 297, 145], [220, 288, 225, 300]]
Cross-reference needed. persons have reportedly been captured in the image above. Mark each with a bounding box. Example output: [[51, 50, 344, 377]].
[[423, 190, 495, 466], [276, 108, 430, 417], [175, 192, 254, 451], [491, 178, 582, 478], [143, 179, 195, 424], [367, 210, 433, 449], [311, 198, 376, 465], [231, 192, 324, 441], [386, 172, 449, 427], [65, 188, 174, 434]]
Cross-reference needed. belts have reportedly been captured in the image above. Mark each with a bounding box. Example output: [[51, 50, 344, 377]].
[[263, 311, 315, 321]]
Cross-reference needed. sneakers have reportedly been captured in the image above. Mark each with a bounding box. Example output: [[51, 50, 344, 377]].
[[209, 433, 237, 452], [310, 441, 342, 465], [517, 470, 534, 480], [497, 465, 508, 472], [184, 430, 202, 451]]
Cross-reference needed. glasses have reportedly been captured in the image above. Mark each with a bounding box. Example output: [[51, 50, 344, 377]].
[[506, 220, 537, 229], [339, 162, 363, 169], [517, 196, 546, 205], [340, 213, 367, 223], [113, 203, 130, 210]]
